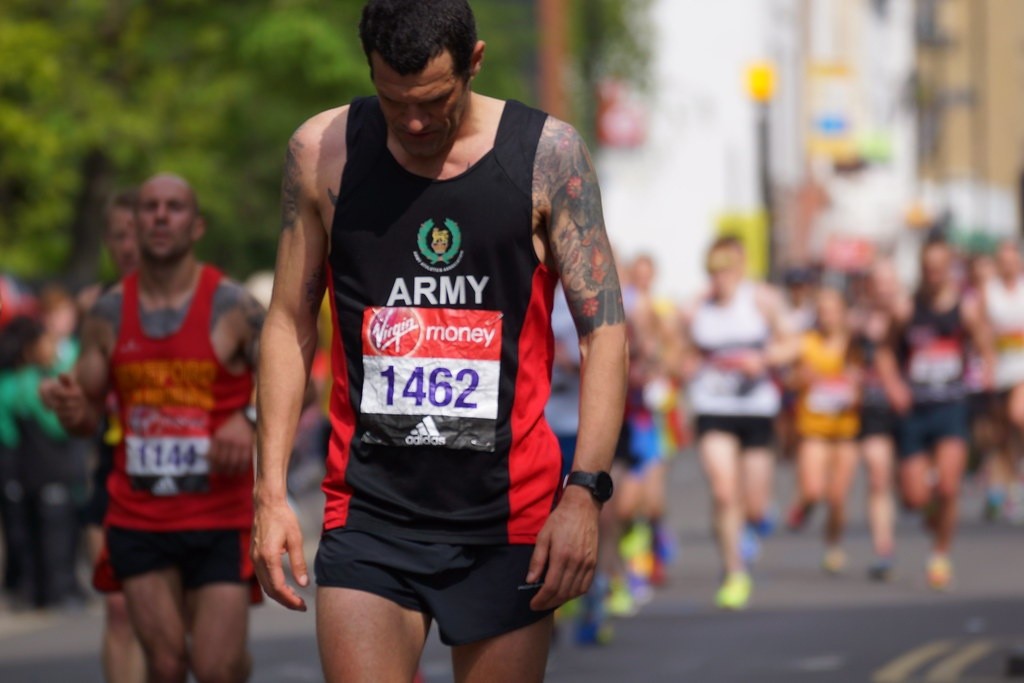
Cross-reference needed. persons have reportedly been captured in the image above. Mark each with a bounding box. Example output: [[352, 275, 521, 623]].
[[543, 230, 1023, 643], [254, 0, 629, 683], [0, 172, 332, 683]]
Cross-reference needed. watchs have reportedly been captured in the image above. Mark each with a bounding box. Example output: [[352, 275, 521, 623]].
[[243, 404, 257, 425], [563, 470, 614, 503]]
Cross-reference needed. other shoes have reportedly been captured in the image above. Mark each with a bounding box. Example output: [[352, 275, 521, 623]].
[[713, 569, 753, 609], [742, 526, 757, 564], [923, 549, 953, 587], [868, 549, 894, 579], [785, 499, 809, 529], [823, 543, 844, 572], [750, 512, 777, 539], [980, 482, 1006, 520]]
